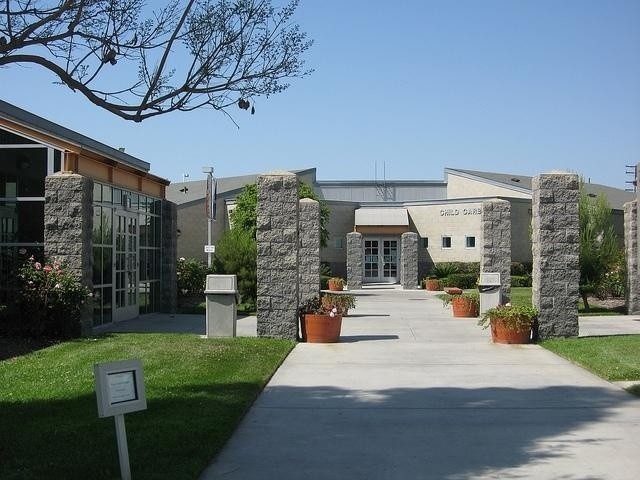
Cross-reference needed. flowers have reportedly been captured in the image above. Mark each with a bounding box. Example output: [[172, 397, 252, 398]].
[[11, 255, 99, 321]]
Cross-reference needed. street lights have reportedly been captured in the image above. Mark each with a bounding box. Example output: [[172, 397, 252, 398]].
[[202, 166, 217, 269]]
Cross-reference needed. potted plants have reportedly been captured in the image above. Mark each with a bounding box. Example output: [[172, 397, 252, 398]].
[[479, 303, 539, 345], [443, 293, 479, 318], [325, 277, 347, 291], [299, 296, 342, 344], [422, 277, 441, 290]]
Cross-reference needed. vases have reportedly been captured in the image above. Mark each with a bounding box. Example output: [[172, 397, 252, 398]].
[[19, 313, 82, 338]]
